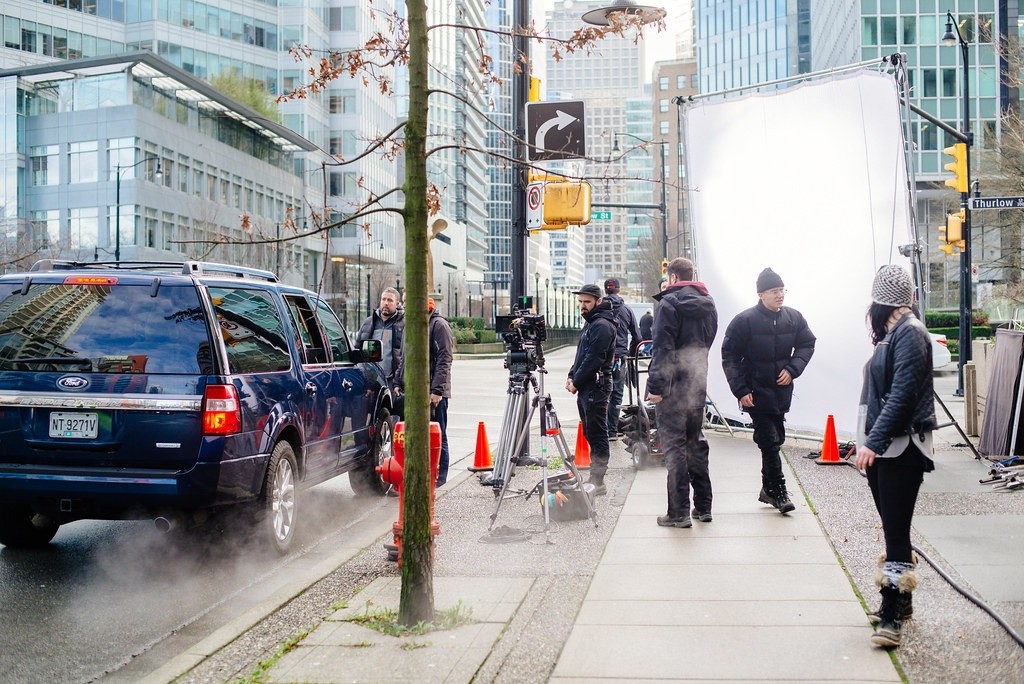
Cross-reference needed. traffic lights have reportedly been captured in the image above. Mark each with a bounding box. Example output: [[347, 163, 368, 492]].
[[938, 225, 953, 255], [943, 143, 968, 193], [945, 215, 961, 244], [661, 261, 669, 279]]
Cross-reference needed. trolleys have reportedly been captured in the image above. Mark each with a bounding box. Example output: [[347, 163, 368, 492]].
[[616, 340, 665, 471]]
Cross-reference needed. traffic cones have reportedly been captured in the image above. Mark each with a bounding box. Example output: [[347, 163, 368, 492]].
[[466, 421, 494, 472], [814, 414, 848, 466], [569, 421, 592, 470]]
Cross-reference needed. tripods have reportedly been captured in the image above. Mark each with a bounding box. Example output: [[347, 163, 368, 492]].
[[482, 368, 598, 545]]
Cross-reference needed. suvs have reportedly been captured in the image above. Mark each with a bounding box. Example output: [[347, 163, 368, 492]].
[[0, 259, 393, 557]]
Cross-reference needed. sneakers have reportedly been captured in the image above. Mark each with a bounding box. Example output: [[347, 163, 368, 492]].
[[657, 514, 692, 528], [693, 508, 711, 521]]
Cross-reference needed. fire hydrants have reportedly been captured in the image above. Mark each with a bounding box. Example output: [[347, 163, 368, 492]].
[[374, 420, 443, 569]]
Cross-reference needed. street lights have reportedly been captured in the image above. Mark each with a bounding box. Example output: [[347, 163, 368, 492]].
[[365, 266, 373, 318], [357, 239, 385, 332], [610, 130, 691, 260], [567, 290, 572, 328], [508, 0, 668, 467], [941, 9, 982, 398], [553, 281, 559, 328], [435, 268, 494, 328], [93, 157, 164, 266], [276, 216, 309, 278], [534, 272, 541, 315], [545, 278, 551, 328], [572, 294, 577, 329], [395, 272, 401, 292], [560, 286, 566, 329]]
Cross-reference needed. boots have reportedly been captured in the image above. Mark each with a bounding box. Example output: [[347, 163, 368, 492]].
[[871, 562, 916, 646], [771, 483, 795, 513], [870, 550, 919, 622], [760, 476, 778, 508]]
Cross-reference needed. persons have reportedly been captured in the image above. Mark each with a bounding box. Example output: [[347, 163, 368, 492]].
[[857, 264, 938, 647], [354, 287, 407, 418], [658, 278, 669, 295], [392, 285, 454, 486], [565, 284, 622, 498], [595, 278, 642, 441], [640, 308, 654, 345], [644, 257, 718, 528], [721, 267, 817, 513]]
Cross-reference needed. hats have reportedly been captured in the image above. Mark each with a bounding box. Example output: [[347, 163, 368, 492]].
[[757, 268, 784, 293], [647, 308, 652, 313], [604, 278, 620, 289], [872, 265, 912, 309], [572, 284, 601, 298]]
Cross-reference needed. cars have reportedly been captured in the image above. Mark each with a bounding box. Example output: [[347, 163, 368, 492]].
[[928, 331, 951, 368]]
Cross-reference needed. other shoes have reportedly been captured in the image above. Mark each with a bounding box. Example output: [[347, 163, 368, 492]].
[[609, 432, 618, 441], [592, 485, 607, 496], [435, 480, 446, 488], [641, 352, 646, 356]]
